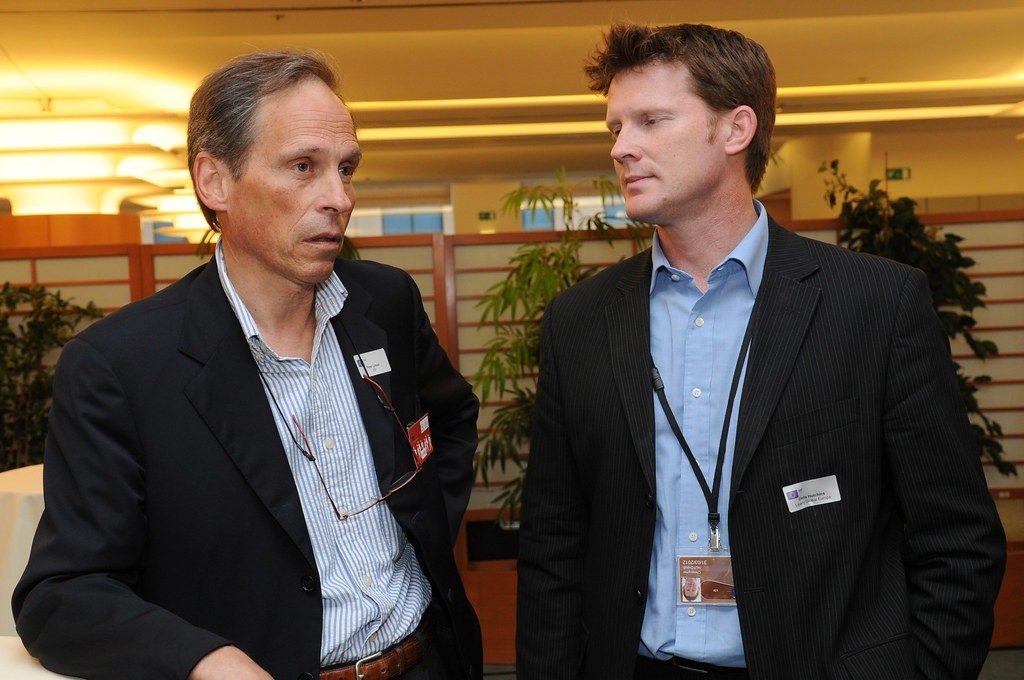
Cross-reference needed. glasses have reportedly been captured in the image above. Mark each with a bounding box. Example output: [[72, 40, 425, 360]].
[[293, 374, 423, 521]]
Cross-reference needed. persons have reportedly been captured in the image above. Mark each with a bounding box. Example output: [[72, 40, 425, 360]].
[[516, 23, 1007, 680], [683, 578, 700, 602], [12, 54, 484, 680]]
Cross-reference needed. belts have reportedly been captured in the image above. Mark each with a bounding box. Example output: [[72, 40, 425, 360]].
[[319, 617, 432, 680]]
